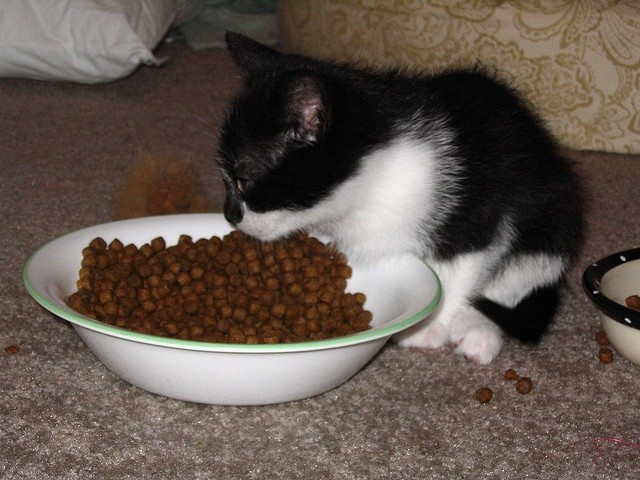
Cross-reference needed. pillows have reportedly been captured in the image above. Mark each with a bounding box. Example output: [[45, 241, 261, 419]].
[[282, 0, 639, 159], [0, 1, 185, 84]]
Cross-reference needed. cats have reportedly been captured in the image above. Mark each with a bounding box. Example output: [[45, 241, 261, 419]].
[[210, 28, 598, 365]]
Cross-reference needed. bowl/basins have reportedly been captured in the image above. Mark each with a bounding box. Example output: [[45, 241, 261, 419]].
[[19, 210, 444, 408], [580, 247, 640, 367]]
